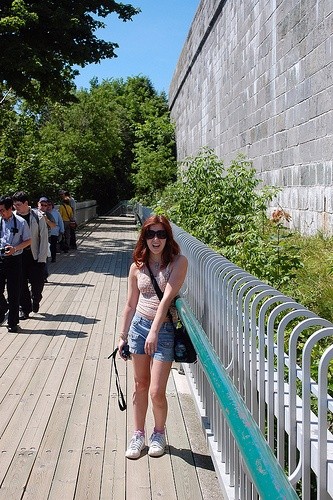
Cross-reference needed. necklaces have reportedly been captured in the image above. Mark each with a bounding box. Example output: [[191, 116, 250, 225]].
[[154, 261, 160, 269]]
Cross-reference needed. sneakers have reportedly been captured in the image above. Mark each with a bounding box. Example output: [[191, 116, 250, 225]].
[[148, 432, 166, 457], [125, 435, 145, 459]]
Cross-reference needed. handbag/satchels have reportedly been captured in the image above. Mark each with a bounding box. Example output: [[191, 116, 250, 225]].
[[173, 325, 196, 363], [70, 218, 75, 227]]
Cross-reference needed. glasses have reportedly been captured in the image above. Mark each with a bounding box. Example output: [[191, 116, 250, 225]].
[[48, 204, 52, 206], [145, 229, 167, 239], [40, 204, 47, 207]]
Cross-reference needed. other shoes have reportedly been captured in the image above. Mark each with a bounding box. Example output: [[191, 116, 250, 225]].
[[51, 258, 56, 262], [0, 307, 8, 323], [18, 309, 29, 320], [32, 299, 39, 313], [7, 323, 17, 332]]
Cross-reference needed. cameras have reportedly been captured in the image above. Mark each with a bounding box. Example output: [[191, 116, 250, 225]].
[[0, 246, 10, 254], [122, 344, 132, 361]]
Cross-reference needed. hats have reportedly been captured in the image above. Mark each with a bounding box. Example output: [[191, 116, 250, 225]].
[[40, 197, 48, 203]]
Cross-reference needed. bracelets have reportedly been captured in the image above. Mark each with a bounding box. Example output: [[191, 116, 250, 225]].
[[14, 247, 17, 252], [46, 219, 50, 223], [120, 332, 128, 339]]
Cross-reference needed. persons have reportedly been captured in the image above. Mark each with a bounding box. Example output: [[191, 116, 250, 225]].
[[0, 194, 32, 332], [56, 192, 77, 254], [36, 196, 56, 284], [11, 191, 48, 320], [118, 215, 188, 459], [46, 199, 64, 263]]
